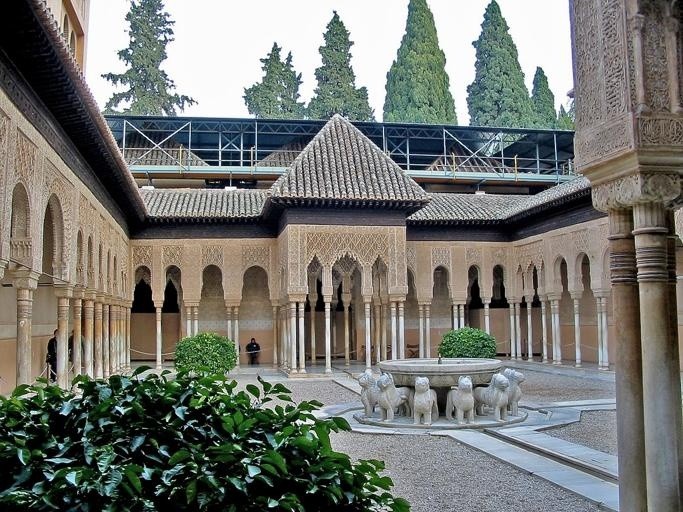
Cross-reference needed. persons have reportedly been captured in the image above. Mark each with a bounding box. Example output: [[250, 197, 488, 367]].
[[47, 329, 58, 383], [246, 337, 260, 365], [67, 330, 73, 369]]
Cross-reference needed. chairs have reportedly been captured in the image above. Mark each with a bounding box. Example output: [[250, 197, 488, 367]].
[[246, 350, 260, 365]]
